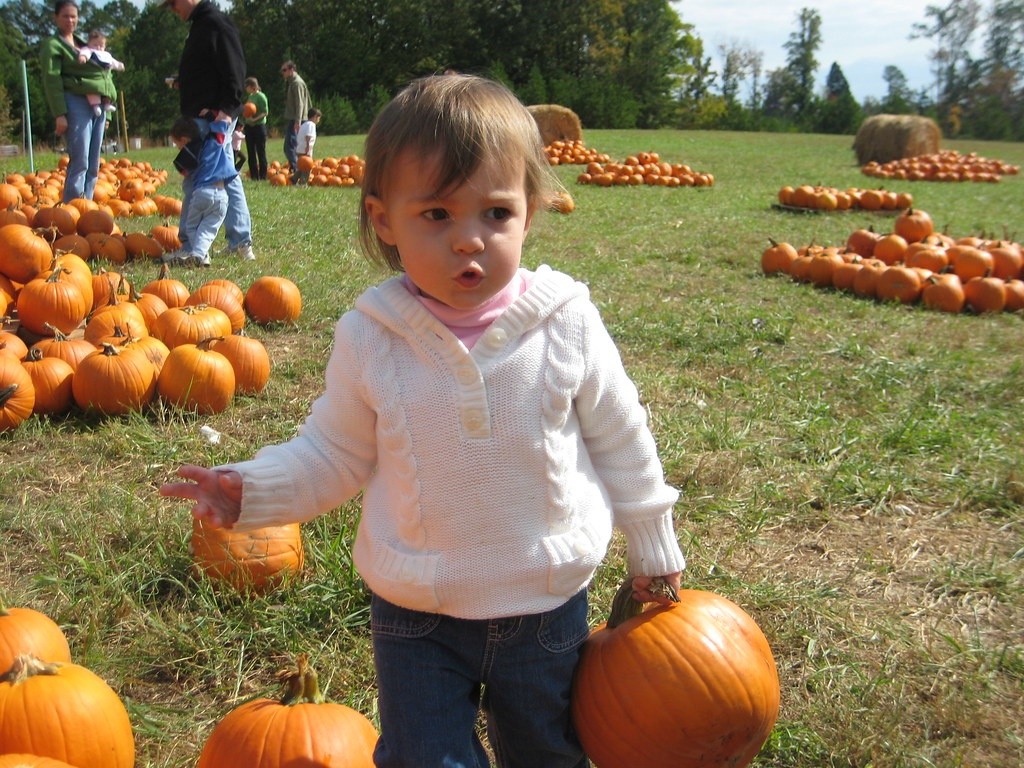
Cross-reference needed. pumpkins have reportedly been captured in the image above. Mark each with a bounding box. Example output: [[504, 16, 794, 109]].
[[573, 577, 779, 768], [190, 512, 304, 589], [242, 101, 256, 117], [194, 658, 379, 768], [245, 153, 367, 187], [540, 138, 715, 214], [761, 149, 1024, 314], [0, 156, 301, 432], [0, 604, 135, 768]]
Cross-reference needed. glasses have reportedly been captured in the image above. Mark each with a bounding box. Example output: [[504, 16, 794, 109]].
[[168, 0, 175, 8], [283, 68, 291, 72], [245, 84, 249, 89]]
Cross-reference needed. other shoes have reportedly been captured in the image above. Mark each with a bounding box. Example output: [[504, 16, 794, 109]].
[[168, 255, 203, 267]]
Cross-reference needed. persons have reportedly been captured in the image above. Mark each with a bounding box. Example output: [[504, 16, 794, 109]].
[[157, 72, 690, 768], [242, 76, 268, 181], [40, 0, 118, 205], [290, 107, 321, 184], [280, 59, 312, 174], [232, 121, 246, 171], [160, 0, 256, 261], [171, 102, 238, 267], [79, 29, 125, 117]]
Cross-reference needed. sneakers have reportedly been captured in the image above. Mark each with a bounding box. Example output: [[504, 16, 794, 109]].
[[213, 242, 255, 263], [161, 249, 211, 267]]
